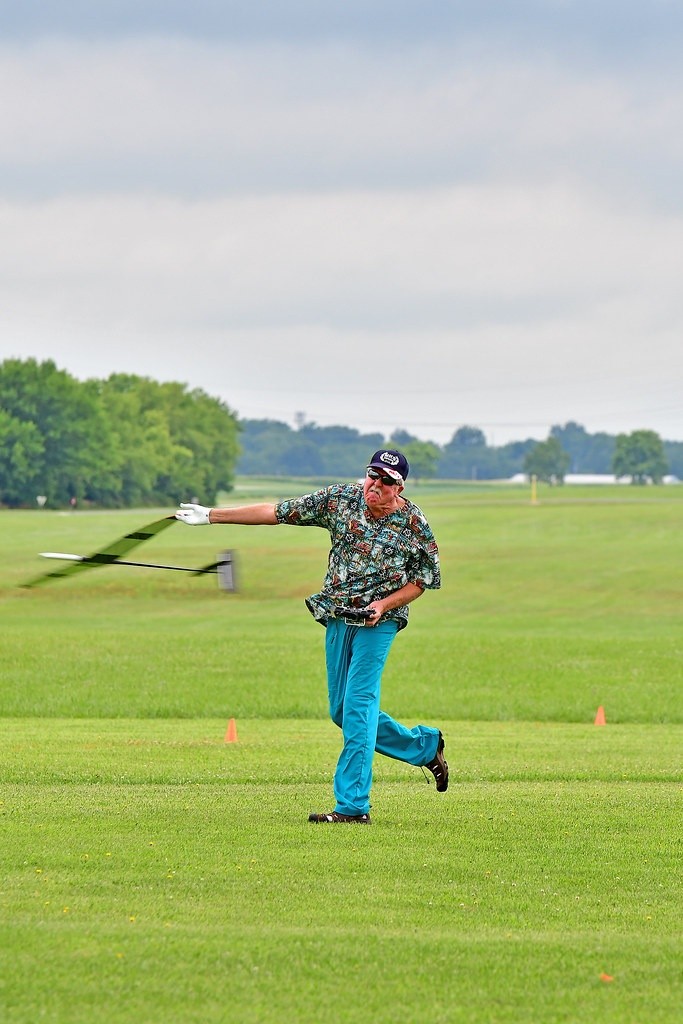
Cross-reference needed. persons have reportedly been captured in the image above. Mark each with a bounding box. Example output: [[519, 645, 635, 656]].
[[174, 448, 449, 823]]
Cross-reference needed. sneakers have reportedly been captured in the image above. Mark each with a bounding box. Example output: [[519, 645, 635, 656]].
[[309, 811, 372, 824], [424, 730, 448, 792]]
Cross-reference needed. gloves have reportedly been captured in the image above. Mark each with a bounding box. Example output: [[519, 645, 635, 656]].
[[176, 503, 214, 525]]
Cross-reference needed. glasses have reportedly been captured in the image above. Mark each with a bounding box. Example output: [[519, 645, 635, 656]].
[[368, 469, 401, 486]]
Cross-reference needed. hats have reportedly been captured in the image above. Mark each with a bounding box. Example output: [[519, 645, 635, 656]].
[[366, 449, 409, 483]]
